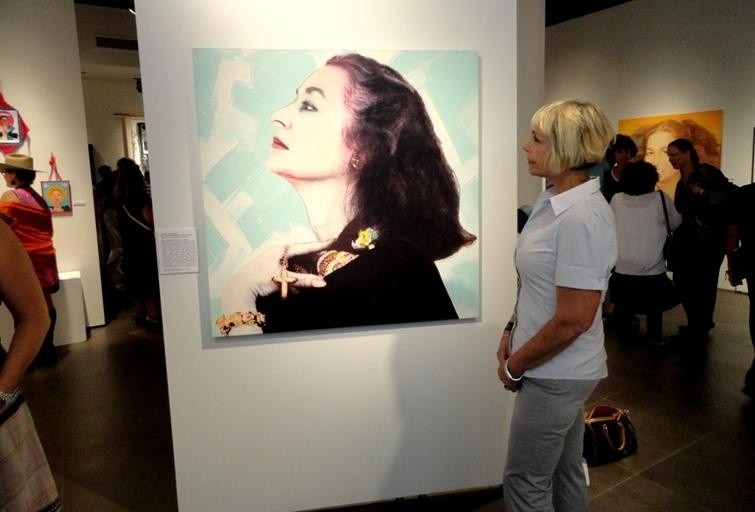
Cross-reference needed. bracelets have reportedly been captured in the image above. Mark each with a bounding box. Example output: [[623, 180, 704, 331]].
[[502, 333, 510, 338], [216, 311, 266, 335], [503, 357, 526, 382], [0, 384, 23, 402]]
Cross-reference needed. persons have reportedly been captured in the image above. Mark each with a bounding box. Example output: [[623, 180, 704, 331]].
[[0, 116, 14, 140], [142, 169, 151, 198], [112, 157, 160, 323], [667, 138, 726, 344], [0, 153, 60, 373], [46, 184, 66, 212], [628, 119, 721, 201], [220, 53, 477, 334], [603, 134, 639, 206], [497, 98, 619, 512], [609, 158, 683, 314], [727, 182, 755, 401], [0, 217, 62, 512], [0, 128, 18, 139], [96, 165, 112, 279]]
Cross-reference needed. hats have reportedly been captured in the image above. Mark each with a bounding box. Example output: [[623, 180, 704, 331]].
[[0, 152, 47, 174]]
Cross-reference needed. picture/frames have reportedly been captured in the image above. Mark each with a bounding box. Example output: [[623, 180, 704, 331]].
[[122, 111, 149, 188]]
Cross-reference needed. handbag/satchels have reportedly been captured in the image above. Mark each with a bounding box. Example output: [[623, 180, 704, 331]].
[[659, 191, 691, 275], [582, 403, 638, 468]]
